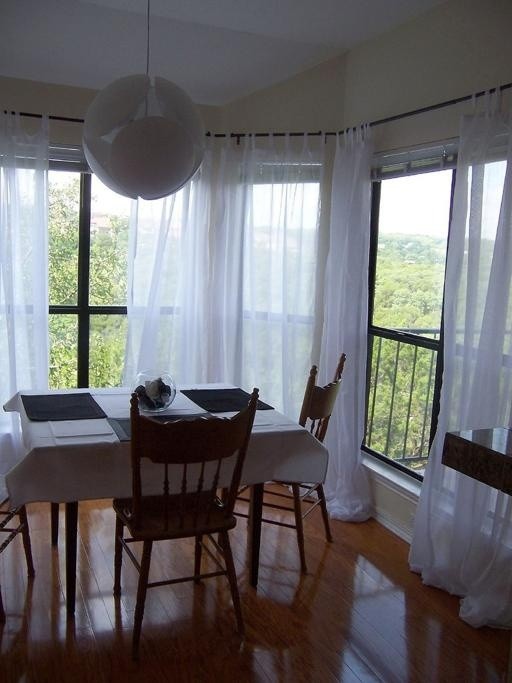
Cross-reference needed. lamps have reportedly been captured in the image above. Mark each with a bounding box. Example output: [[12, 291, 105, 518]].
[[80, 0, 205, 199]]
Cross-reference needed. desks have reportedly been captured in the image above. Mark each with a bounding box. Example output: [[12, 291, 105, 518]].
[[0, 384, 311, 617]]
